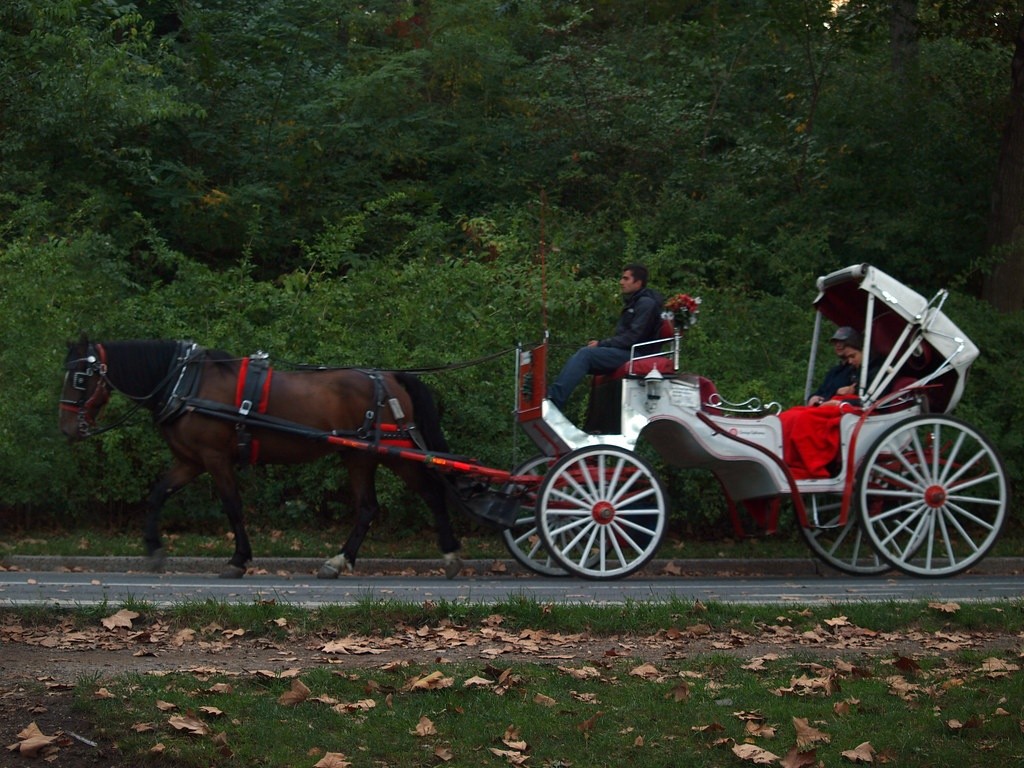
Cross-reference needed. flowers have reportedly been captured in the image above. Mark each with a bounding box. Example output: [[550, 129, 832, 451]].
[[662, 292, 703, 330]]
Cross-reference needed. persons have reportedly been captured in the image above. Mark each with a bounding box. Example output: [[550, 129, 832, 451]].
[[548, 264, 666, 414], [807, 326, 859, 406], [783, 335, 893, 480]]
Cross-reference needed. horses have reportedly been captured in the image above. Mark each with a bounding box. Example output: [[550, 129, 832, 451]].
[[57, 332, 463, 581]]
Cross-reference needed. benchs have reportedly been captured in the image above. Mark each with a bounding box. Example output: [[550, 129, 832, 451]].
[[681, 374, 784, 460], [839, 376, 931, 462], [593, 317, 684, 389]]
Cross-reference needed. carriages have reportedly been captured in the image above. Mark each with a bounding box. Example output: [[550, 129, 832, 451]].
[[57, 258, 1010, 583]]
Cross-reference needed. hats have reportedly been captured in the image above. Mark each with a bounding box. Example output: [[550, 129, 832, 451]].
[[829, 327, 858, 341]]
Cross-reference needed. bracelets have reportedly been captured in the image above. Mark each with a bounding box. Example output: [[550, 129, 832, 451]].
[[840, 402, 852, 408]]
